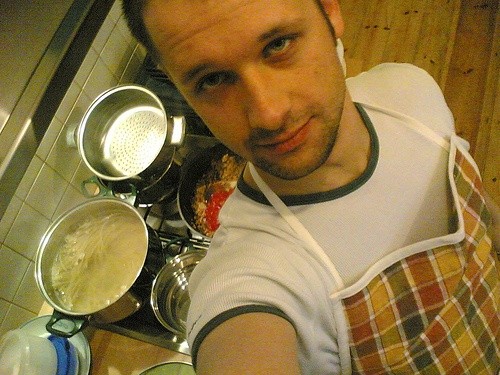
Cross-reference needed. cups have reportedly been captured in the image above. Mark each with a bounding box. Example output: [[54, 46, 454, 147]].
[[0, 329, 57, 375]]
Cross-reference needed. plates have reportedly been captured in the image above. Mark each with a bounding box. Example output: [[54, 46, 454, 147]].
[[139, 361, 196, 375], [17, 315, 91, 375]]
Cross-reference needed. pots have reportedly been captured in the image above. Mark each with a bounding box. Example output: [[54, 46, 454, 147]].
[[34, 182, 166, 338], [177, 141, 248, 250], [66, 82, 190, 208]]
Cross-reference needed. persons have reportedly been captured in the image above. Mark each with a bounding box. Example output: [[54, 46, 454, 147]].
[[121, 0, 500, 375]]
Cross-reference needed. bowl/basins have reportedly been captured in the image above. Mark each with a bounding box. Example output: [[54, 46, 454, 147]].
[[47, 335, 79, 375], [150, 248, 209, 339]]
